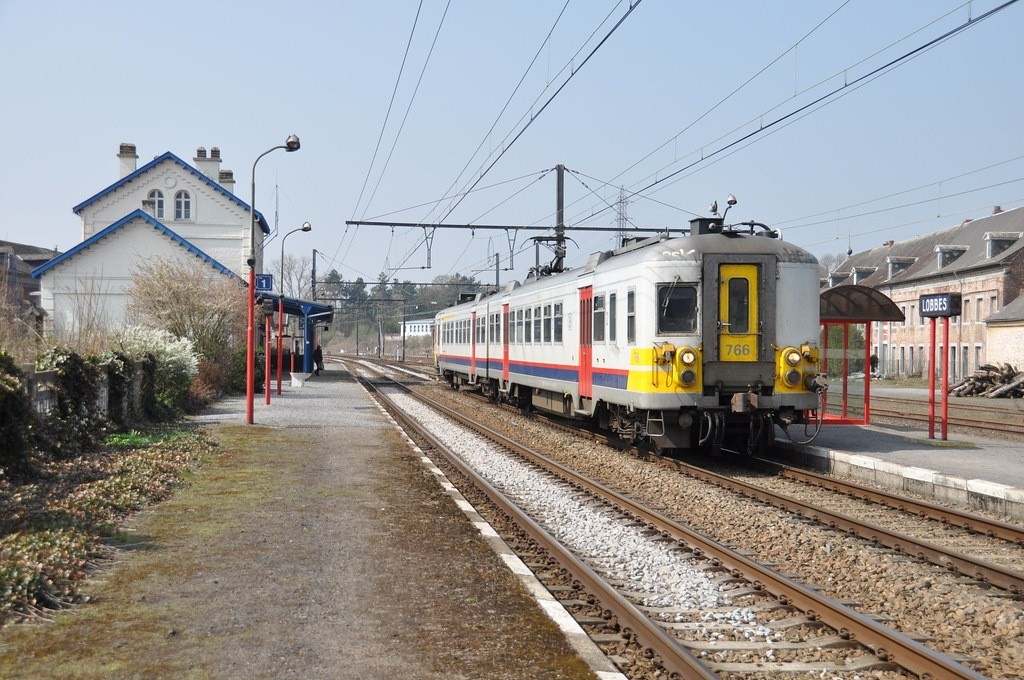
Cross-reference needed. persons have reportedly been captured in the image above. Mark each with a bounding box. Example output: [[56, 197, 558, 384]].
[[313, 345, 322, 376], [295, 340, 299, 357], [870, 354, 878, 373]]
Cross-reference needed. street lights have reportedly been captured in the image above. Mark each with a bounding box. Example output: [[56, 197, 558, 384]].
[[246, 131, 301, 424], [276, 221, 312, 398]]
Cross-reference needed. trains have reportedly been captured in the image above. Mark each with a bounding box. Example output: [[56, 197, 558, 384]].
[[434, 192, 831, 463]]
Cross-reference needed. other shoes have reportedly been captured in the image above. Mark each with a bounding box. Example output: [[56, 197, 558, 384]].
[[314, 371, 320, 376]]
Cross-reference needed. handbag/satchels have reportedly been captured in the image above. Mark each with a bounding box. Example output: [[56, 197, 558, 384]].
[[318, 361, 324, 370]]
